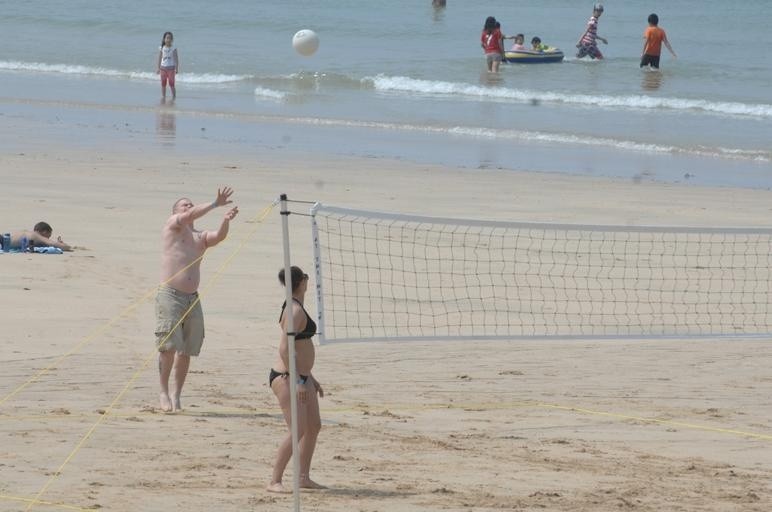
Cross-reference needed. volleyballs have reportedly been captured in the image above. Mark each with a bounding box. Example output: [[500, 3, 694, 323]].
[[293, 30, 319, 56]]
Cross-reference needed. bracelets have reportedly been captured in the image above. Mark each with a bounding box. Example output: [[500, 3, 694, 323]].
[[296, 379, 304, 384], [211, 200, 220, 209]]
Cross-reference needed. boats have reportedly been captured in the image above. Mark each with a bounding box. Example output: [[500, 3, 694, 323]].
[[499, 43, 566, 66]]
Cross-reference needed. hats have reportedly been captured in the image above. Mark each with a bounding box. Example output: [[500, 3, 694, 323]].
[[595, 3, 604, 11]]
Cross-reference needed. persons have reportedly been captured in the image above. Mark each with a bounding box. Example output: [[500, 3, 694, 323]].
[[511, 33, 531, 51], [638, 13, 677, 73], [480, 16, 505, 73], [0, 221, 73, 254], [264, 265, 329, 493], [531, 36, 557, 53], [430, 1, 449, 22], [495, 21, 518, 62], [575, 3, 607, 60], [155, 31, 180, 105], [152, 185, 241, 415]]
